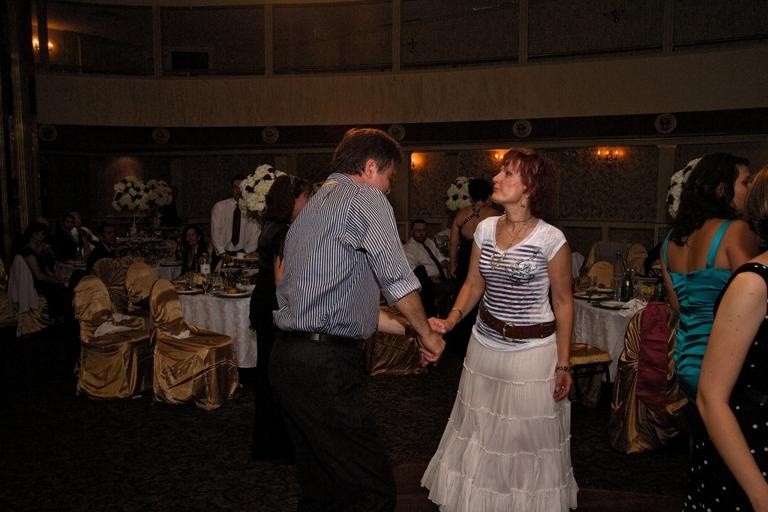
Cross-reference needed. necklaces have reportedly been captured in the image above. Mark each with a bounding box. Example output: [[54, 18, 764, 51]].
[[489, 216, 537, 270]]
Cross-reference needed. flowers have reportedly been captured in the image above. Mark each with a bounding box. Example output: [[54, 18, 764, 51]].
[[663, 153, 699, 216], [108, 175, 149, 214], [142, 178, 173, 207], [443, 174, 476, 212], [238, 163, 288, 216]]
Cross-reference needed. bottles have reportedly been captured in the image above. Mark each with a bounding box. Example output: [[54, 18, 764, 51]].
[[613, 249, 633, 301], [231, 257, 242, 278], [656, 274, 665, 298], [220, 253, 229, 280], [192, 247, 211, 280]]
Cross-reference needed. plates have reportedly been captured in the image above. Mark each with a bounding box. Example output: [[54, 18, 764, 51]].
[[177, 287, 201, 293], [216, 293, 250, 297], [574, 287, 625, 307]]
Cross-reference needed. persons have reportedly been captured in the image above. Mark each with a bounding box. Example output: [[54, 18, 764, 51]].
[[17, 211, 118, 372], [175, 224, 209, 282], [643, 152, 767, 512], [269, 127, 446, 512], [421, 147, 580, 512], [405, 179, 503, 366], [211, 175, 315, 465]]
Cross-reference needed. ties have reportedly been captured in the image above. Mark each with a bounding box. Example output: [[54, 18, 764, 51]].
[[231, 203, 241, 247], [421, 241, 446, 278]]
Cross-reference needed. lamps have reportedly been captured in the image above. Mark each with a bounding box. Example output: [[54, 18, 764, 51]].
[[595, 148, 620, 169], [31, 35, 54, 53]]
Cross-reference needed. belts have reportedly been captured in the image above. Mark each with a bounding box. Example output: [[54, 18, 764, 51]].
[[274, 330, 367, 351], [479, 298, 556, 343]]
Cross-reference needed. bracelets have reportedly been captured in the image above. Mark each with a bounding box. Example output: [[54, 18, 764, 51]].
[[451, 308, 464, 323], [554, 366, 572, 373]]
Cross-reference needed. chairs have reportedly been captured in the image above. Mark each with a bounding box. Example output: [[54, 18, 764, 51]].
[[0, 218, 683, 359], [148, 276, 240, 415], [568, 336, 612, 420], [121, 261, 156, 319], [622, 301, 684, 454], [73, 273, 147, 399]]
